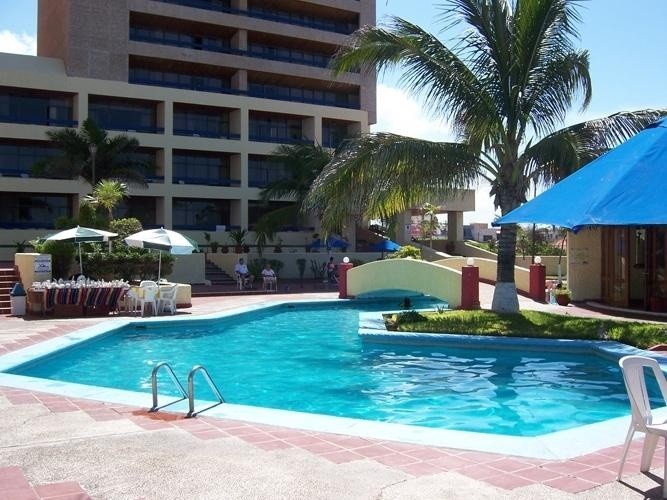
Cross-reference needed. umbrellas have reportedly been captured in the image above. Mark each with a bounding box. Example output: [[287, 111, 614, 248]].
[[308, 235, 352, 260], [46, 224, 119, 276], [123, 225, 200, 283], [489, 116, 666, 235], [368, 239, 403, 260]]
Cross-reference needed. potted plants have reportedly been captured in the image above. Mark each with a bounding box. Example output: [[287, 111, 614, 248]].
[[551, 285, 574, 307], [198, 226, 285, 254]]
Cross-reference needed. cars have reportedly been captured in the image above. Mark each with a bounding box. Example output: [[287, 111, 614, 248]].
[[369, 224, 388, 236]]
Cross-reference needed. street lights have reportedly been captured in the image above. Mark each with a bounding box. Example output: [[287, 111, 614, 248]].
[[89, 142, 98, 191]]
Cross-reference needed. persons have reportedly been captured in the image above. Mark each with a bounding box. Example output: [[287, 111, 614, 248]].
[[260, 262, 277, 291], [326, 256, 338, 282], [234, 257, 255, 290]]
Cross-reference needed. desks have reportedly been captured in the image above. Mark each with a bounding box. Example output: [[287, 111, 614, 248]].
[[27, 285, 124, 316]]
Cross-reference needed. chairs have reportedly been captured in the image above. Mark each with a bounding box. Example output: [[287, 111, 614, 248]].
[[135, 280, 181, 317], [616, 355, 665, 500], [261, 272, 278, 292], [234, 270, 252, 289]]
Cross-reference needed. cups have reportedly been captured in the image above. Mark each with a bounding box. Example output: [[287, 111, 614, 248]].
[[31, 277, 120, 290]]
